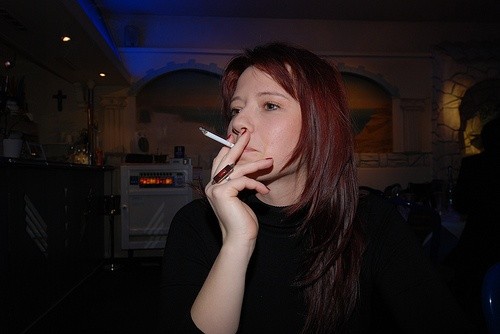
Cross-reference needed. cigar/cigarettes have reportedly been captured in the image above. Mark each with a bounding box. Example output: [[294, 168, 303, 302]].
[[198, 127, 235, 148]]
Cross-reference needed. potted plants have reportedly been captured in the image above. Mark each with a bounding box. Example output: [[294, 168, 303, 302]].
[[1, 112, 24, 158]]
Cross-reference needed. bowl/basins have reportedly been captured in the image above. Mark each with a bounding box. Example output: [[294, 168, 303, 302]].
[[3, 139, 24, 158]]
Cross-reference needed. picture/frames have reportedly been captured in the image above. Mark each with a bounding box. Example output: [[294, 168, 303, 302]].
[[27, 143, 45, 160], [22, 132, 41, 162]]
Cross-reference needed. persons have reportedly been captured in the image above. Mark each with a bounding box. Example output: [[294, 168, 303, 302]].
[[161, 39, 473, 334], [456, 118, 500, 325]]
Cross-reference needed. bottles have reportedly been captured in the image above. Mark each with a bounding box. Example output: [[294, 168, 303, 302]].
[[447, 166, 456, 212]]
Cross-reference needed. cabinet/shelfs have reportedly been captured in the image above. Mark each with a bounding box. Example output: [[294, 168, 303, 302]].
[[0, 164, 112, 332]]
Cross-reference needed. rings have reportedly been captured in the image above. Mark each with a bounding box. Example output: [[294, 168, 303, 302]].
[[212, 162, 235, 185]]
